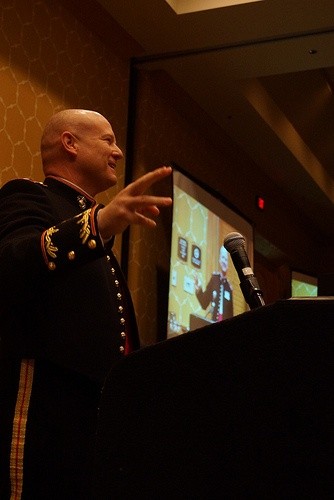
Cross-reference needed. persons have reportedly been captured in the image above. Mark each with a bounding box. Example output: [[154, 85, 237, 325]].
[[0, 108, 172, 500], [192, 245, 234, 323]]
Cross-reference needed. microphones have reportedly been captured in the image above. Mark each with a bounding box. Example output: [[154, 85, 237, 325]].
[[212, 290, 217, 307], [223, 232, 265, 310]]
